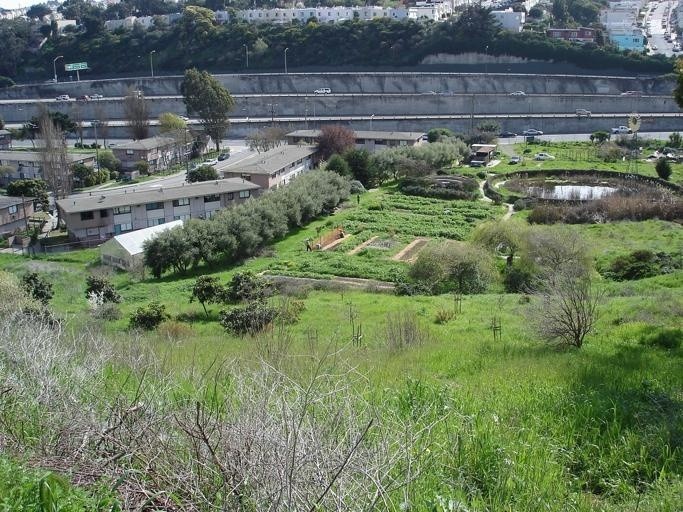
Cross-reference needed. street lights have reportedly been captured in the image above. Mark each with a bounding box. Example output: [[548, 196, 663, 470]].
[[52, 56, 63, 80], [267, 103, 278, 126], [242, 44, 248, 69], [241, 109, 250, 133], [485, 45, 488, 72], [149, 50, 155, 77], [284, 48, 288, 73], [368, 114, 375, 131]]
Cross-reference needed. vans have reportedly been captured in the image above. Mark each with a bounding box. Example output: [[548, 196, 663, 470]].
[[313, 87, 331, 95]]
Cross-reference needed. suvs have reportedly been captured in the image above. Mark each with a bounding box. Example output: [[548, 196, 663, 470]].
[[575, 109, 590, 117]]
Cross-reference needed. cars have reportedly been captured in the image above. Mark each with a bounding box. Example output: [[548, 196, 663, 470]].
[[509, 90, 526, 97], [521, 128, 543, 136], [217, 153, 229, 161], [509, 156, 521, 164], [202, 159, 217, 167], [130, 91, 140, 98], [621, 91, 639, 97], [497, 131, 517, 137], [55, 94, 103, 101], [645, 1, 682, 52], [534, 153, 555, 162]]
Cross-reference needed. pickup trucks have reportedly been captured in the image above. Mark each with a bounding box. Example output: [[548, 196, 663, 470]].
[[611, 125, 632, 134]]
